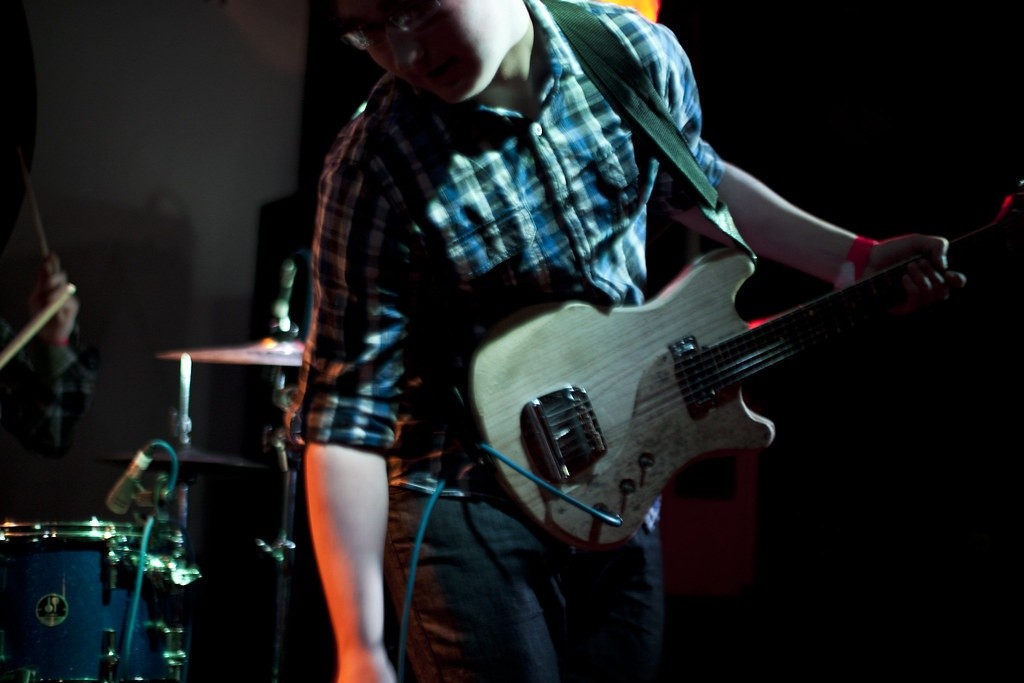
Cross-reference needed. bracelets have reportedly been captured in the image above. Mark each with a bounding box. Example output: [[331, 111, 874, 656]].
[[846, 234, 876, 280]]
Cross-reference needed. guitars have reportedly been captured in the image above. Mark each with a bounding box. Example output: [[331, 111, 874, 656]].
[[468, 177, 1024, 552]]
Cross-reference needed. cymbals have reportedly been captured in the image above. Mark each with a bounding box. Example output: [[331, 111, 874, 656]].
[[95, 445, 272, 478], [152, 341, 311, 369]]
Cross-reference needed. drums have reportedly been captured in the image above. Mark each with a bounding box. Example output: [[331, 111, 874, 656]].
[[0, 516, 194, 683]]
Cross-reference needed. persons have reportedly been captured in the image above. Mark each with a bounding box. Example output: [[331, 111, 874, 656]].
[[287, 0, 949, 683], [0, 250, 100, 460]]
